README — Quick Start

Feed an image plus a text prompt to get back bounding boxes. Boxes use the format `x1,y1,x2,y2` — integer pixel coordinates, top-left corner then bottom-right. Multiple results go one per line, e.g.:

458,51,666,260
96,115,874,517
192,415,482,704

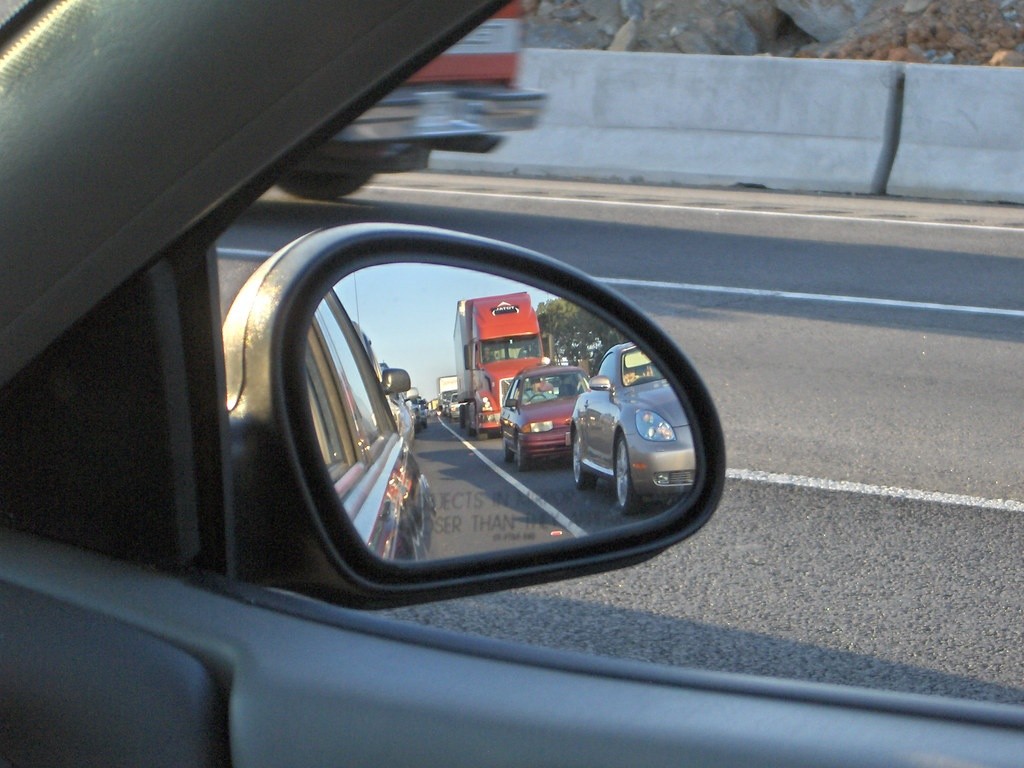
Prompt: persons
624,365,653,384
482,348,495,363
523,377,558,405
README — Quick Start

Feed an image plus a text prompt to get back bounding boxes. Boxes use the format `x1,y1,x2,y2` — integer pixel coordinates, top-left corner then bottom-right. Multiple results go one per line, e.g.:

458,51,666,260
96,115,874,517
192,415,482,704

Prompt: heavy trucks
453,290,546,439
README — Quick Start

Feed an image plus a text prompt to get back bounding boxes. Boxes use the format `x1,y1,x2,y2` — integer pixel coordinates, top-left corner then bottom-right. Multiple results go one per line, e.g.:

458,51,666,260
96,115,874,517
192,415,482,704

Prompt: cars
352,321,429,451
568,343,696,517
304,283,439,564
443,394,467,423
498,367,594,471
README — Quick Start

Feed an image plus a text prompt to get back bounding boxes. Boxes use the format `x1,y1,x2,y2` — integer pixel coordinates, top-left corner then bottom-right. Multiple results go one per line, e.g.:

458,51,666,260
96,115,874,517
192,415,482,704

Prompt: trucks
436,375,457,412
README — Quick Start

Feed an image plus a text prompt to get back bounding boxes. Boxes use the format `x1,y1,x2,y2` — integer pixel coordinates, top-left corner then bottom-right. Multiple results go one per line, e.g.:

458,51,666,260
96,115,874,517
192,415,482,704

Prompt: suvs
278,1,552,199
